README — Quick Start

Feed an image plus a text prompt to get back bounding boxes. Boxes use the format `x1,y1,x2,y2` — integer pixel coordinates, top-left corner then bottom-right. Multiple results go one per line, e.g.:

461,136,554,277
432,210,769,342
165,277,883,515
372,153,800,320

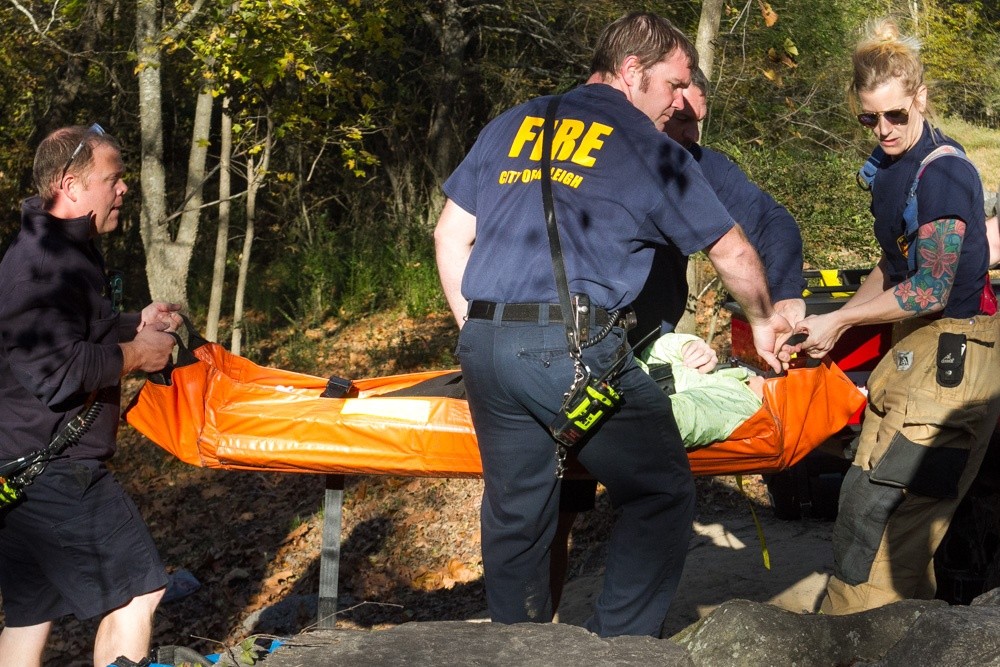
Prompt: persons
432,10,805,639
792,19,1000,616
1,127,184,667
365,332,766,448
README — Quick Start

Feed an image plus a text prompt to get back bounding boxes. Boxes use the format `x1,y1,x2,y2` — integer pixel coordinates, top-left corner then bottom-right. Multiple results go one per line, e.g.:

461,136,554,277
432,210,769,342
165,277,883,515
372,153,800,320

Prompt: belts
467,300,611,326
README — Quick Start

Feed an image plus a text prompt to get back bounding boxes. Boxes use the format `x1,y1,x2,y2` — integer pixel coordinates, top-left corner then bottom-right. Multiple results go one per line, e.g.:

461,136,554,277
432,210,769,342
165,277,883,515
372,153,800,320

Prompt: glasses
856,91,918,129
59,123,105,189
